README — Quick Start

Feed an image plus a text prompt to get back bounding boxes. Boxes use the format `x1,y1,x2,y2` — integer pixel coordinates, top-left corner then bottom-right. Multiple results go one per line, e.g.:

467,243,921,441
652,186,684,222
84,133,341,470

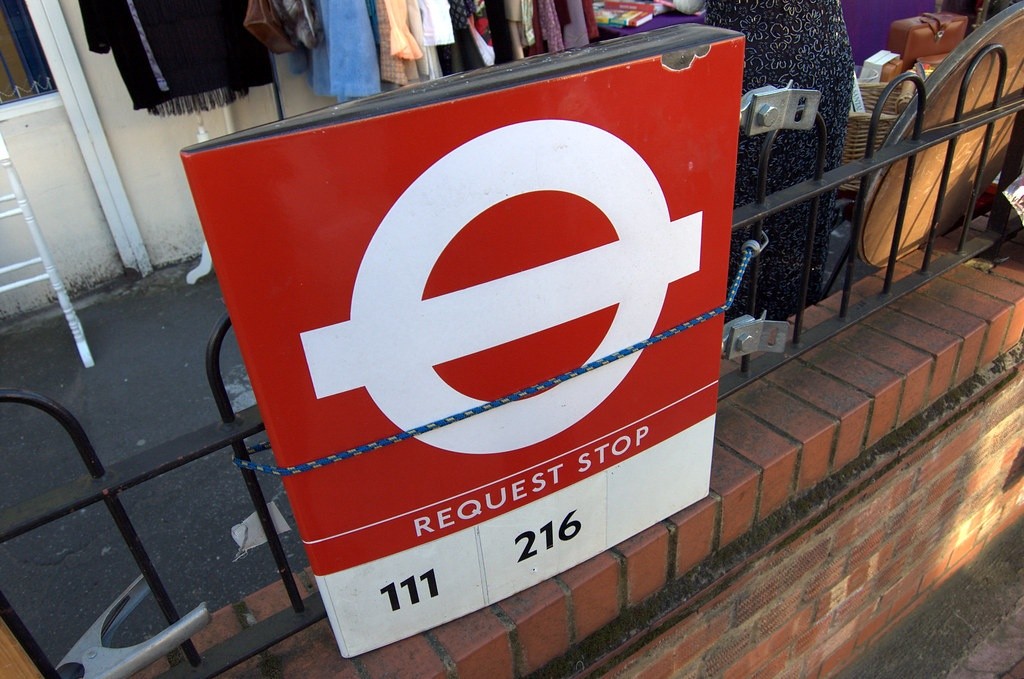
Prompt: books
592,0,706,28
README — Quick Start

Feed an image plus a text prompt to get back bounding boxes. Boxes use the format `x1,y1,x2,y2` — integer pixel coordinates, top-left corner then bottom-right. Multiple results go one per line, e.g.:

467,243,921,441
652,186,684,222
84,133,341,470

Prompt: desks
598,10,705,38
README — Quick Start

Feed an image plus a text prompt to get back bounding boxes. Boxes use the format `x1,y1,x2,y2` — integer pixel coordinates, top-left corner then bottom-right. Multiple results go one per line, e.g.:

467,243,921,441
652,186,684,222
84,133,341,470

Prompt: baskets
838,79,909,195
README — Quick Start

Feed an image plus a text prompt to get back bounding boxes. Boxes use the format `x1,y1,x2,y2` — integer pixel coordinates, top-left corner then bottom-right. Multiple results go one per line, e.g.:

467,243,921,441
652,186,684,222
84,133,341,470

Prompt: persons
672,0,855,321
